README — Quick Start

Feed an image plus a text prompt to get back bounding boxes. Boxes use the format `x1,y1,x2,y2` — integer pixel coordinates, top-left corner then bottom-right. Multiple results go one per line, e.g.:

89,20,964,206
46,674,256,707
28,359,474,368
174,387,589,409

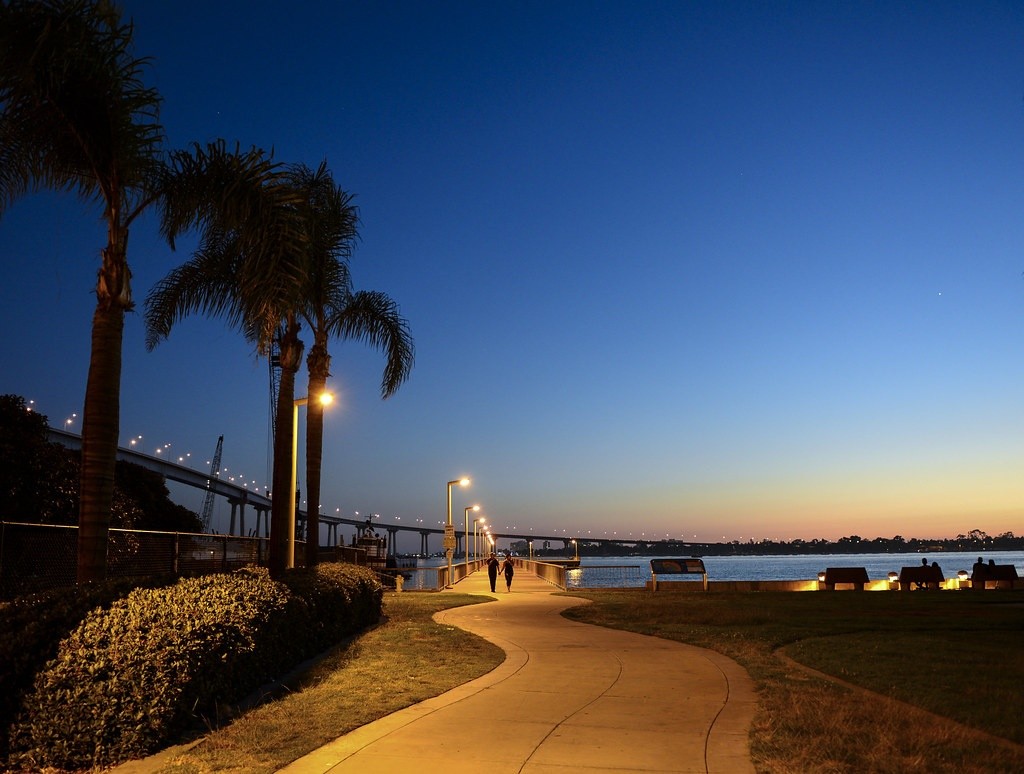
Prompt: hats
488,552,495,555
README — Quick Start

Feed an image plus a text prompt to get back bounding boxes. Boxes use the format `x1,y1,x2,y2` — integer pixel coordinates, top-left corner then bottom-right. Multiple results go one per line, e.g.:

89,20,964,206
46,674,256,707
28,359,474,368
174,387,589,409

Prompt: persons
498,553,514,592
486,552,501,593
971,557,999,589
914,558,939,591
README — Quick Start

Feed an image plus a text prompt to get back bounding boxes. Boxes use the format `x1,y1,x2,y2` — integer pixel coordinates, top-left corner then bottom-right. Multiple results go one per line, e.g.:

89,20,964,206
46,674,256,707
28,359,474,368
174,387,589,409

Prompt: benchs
967,564,1019,589
893,566,945,590
824,567,870,590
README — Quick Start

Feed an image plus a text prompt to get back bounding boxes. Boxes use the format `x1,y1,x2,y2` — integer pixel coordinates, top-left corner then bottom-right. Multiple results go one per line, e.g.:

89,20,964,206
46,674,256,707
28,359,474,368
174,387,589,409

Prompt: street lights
529,541,532,560
572,540,577,560
464,506,480,577
473,517,486,572
478,526,494,572
442,476,469,590
284,393,334,569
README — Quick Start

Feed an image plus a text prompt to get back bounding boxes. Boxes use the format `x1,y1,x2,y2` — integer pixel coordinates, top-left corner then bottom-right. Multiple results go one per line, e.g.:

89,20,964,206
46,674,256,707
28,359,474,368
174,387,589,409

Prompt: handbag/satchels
512,566,515,574
